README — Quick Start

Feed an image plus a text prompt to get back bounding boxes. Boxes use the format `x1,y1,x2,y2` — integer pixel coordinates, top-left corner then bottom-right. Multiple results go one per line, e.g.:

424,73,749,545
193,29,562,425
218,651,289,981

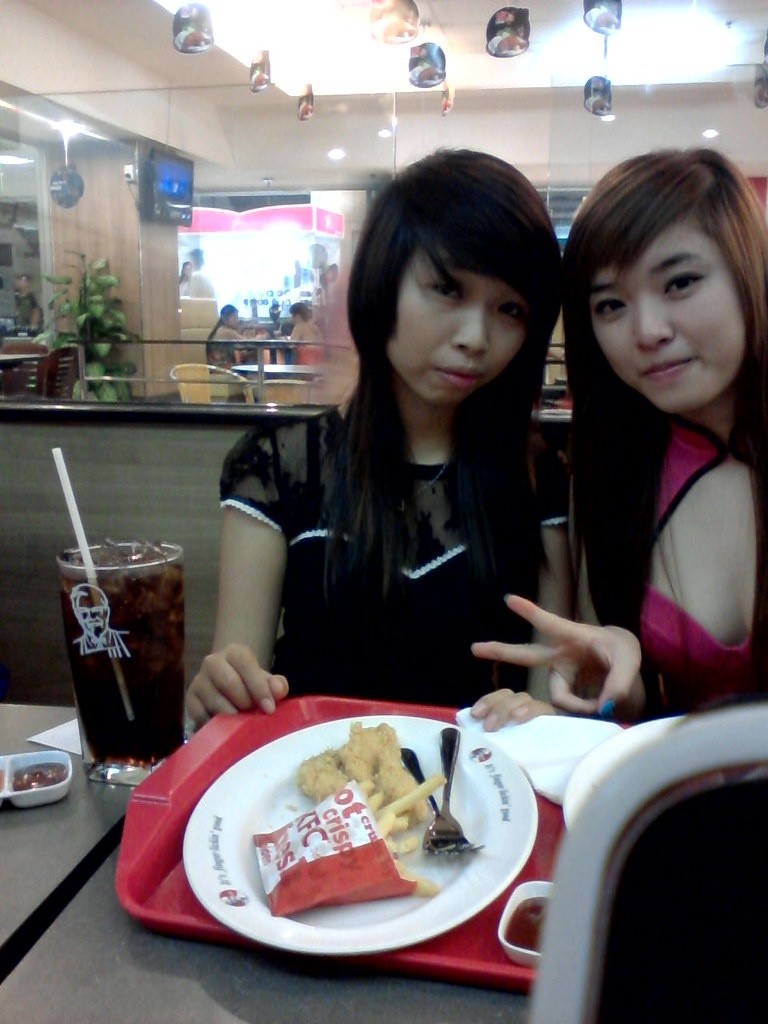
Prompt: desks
0,701,534,1024
232,363,320,379
0,353,47,365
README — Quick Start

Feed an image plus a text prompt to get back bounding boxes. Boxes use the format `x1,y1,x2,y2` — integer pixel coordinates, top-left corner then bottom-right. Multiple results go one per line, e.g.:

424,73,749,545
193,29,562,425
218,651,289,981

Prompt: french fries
361,771,447,897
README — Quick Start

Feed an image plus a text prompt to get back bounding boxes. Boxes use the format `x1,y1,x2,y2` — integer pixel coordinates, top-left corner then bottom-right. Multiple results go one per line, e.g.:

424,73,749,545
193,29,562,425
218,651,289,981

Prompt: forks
401,748,485,852
422,728,465,858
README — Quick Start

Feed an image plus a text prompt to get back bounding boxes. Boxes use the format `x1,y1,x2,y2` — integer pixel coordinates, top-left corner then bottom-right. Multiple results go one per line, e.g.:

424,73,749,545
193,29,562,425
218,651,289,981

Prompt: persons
180,149,572,733
15,275,38,324
470,141,768,717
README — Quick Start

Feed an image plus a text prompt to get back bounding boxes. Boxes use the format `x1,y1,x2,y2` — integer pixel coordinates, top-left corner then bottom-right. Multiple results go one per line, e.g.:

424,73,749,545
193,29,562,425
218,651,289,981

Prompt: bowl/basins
0,750,72,808
498,882,552,966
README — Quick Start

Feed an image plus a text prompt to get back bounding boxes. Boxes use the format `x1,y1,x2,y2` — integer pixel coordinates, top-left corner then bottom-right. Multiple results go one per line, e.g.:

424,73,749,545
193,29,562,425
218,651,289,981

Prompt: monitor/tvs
140,146,195,227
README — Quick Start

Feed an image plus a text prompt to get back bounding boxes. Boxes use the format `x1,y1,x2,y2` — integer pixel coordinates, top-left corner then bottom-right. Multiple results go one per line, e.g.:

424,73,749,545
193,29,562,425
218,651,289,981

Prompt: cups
56,542,186,785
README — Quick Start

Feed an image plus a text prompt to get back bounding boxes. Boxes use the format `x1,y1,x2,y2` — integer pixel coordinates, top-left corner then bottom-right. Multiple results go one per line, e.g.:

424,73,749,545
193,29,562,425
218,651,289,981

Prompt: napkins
454,703,626,807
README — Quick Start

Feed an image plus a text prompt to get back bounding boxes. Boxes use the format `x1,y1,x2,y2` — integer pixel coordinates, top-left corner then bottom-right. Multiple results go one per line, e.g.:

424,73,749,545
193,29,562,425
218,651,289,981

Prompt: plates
182,716,538,955
563,716,682,826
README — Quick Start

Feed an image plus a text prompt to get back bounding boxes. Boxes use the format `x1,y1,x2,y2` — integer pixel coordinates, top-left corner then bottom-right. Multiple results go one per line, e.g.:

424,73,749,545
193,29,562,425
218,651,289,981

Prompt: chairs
170,364,311,405
0,343,80,399
